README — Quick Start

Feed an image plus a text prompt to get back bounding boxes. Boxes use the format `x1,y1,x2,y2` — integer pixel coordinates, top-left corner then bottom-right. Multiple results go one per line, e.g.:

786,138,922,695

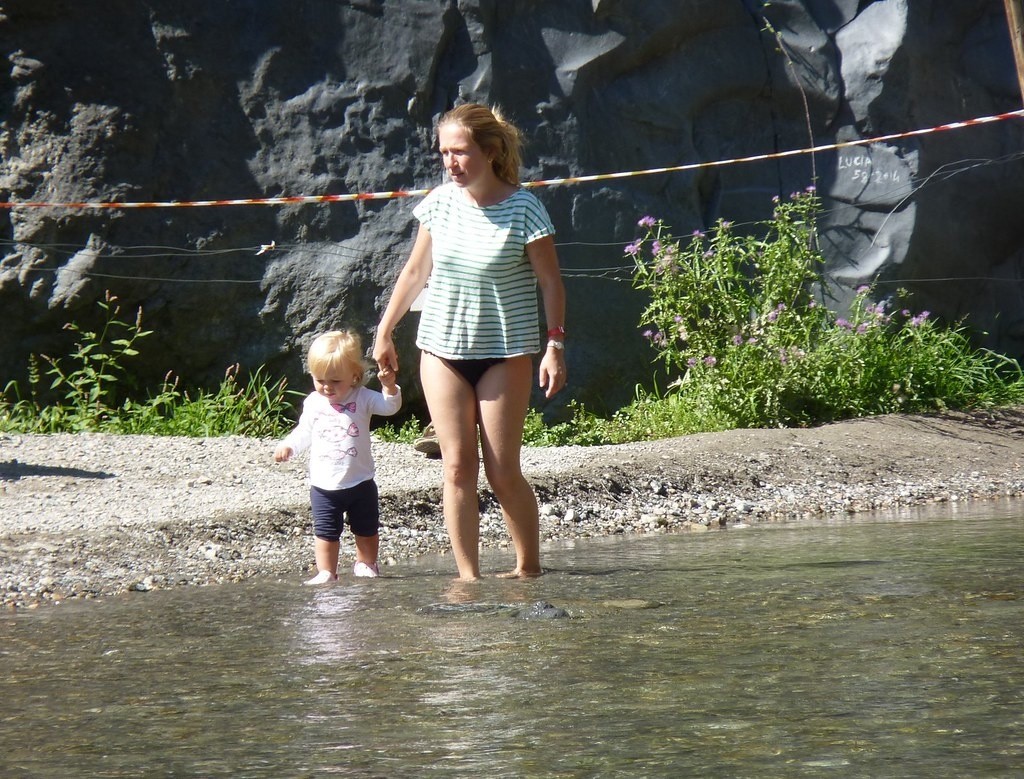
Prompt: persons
274,328,403,586
372,103,567,582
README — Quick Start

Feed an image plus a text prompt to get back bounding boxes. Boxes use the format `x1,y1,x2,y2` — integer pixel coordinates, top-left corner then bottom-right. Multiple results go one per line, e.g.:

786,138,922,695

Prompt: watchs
547,341,565,352
548,325,565,336
